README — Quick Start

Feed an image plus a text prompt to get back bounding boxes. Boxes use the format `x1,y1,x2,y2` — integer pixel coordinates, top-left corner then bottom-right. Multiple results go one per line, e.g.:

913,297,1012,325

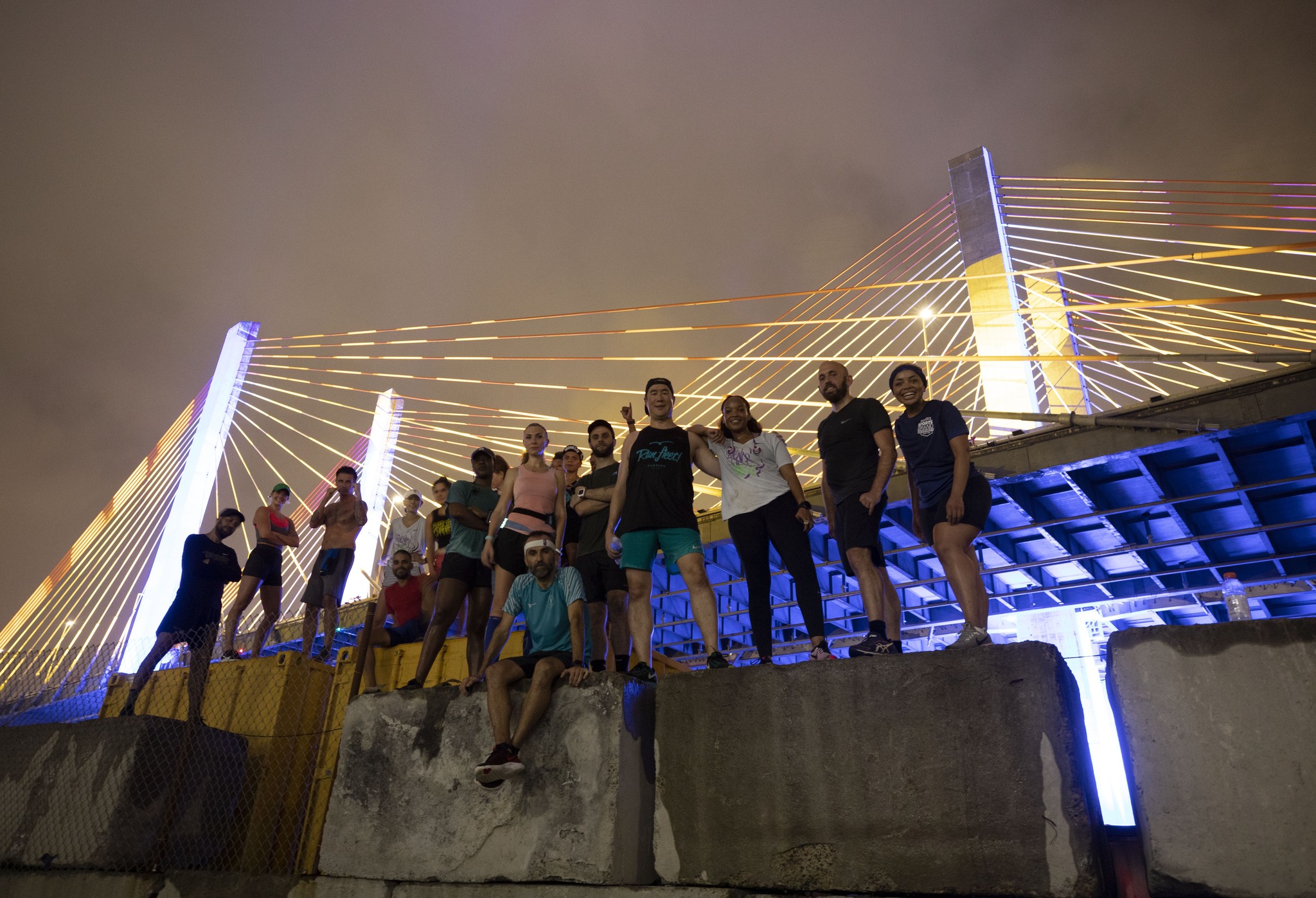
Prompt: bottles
610,537,623,565
1221,572,1252,622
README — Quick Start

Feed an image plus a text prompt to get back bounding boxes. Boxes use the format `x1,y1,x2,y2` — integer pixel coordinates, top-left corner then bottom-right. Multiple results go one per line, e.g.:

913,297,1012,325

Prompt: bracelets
424,557,428,563
555,549,563,556
485,535,494,541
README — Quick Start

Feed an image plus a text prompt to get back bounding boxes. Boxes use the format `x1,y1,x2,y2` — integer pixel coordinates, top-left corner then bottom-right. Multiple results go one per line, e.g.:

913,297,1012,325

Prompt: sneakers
809,635,837,661
310,645,333,664
619,660,658,685
751,656,773,665
392,678,423,692
945,621,994,651
219,649,246,662
848,632,900,658
705,644,736,669
473,741,526,791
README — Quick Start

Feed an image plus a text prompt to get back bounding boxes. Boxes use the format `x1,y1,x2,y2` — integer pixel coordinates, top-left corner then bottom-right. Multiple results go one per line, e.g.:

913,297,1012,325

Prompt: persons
568,419,631,675
380,489,427,590
397,447,500,691
459,530,593,791
589,453,597,473
480,422,567,681
299,465,368,663
423,476,467,638
551,451,567,475
219,483,300,662
604,378,787,678
888,362,992,650
361,548,438,693
557,445,584,567
119,507,245,725
817,362,903,657
686,395,838,663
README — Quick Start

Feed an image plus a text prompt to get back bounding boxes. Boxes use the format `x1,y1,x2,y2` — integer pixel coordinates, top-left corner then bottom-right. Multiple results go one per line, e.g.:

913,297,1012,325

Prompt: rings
804,520,809,525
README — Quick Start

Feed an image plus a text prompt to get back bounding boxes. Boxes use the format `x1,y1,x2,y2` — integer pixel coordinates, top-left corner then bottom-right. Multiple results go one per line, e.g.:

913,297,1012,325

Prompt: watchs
798,501,811,510
578,488,588,500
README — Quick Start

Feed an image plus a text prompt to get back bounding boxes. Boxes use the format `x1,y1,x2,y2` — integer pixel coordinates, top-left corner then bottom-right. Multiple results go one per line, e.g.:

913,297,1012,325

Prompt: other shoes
119,704,135,717
347,687,381,705
186,715,209,728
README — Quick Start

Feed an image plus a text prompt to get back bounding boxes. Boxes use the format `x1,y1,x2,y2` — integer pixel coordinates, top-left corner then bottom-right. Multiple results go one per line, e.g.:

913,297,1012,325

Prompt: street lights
920,309,934,401
373,495,400,595
45,620,74,683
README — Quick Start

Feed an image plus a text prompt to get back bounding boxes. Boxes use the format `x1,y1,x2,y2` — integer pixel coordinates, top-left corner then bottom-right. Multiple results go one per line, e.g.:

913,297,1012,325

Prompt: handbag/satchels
431,678,463,689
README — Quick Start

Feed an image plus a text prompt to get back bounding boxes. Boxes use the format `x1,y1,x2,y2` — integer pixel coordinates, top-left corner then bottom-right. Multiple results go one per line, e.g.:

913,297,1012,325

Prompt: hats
564,445,585,463
587,419,613,435
644,378,673,417
471,447,495,459
405,489,422,501
271,483,291,497
219,508,245,522
